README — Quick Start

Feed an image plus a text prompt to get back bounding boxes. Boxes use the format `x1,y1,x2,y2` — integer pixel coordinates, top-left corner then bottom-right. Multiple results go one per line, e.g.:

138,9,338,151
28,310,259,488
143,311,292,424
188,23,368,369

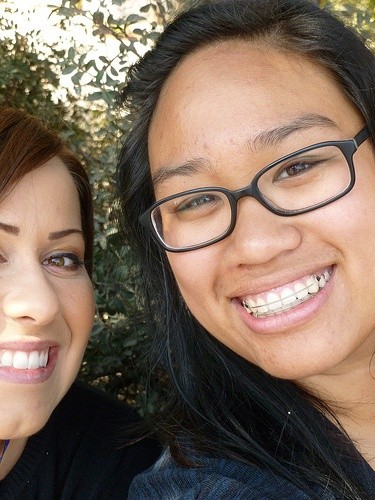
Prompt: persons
0,105,166,500
114,0,375,500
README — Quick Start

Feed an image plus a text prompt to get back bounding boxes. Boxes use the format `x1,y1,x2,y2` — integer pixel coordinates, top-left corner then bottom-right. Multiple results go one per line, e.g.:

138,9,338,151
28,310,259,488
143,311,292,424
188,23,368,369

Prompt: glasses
137,124,375,253
